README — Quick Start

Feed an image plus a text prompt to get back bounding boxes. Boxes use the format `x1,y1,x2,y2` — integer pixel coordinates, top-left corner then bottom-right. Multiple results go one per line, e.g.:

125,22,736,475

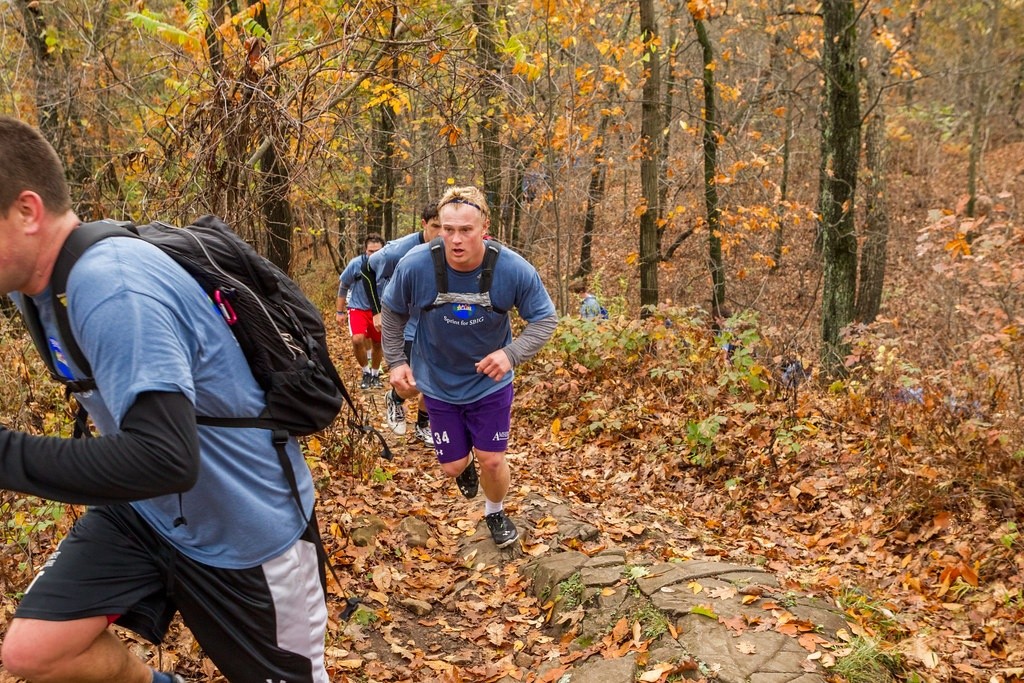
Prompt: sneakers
383,389,407,436
413,421,435,447
483,510,519,549
371,374,383,389
361,371,372,388
456,448,480,500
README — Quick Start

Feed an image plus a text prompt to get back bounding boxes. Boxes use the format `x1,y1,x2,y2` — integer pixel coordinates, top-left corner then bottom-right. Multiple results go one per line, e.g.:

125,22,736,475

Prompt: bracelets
337,311,344,315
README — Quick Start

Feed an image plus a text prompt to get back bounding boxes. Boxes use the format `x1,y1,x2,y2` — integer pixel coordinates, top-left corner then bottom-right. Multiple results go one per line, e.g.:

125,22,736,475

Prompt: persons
335,232,386,388
575,287,602,320
380,186,558,549
367,199,446,447
712,305,739,352
0,118,331,683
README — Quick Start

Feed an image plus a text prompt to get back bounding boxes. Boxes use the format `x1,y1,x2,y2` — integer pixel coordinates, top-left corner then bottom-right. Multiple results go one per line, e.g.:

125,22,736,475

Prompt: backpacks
20,214,393,623
